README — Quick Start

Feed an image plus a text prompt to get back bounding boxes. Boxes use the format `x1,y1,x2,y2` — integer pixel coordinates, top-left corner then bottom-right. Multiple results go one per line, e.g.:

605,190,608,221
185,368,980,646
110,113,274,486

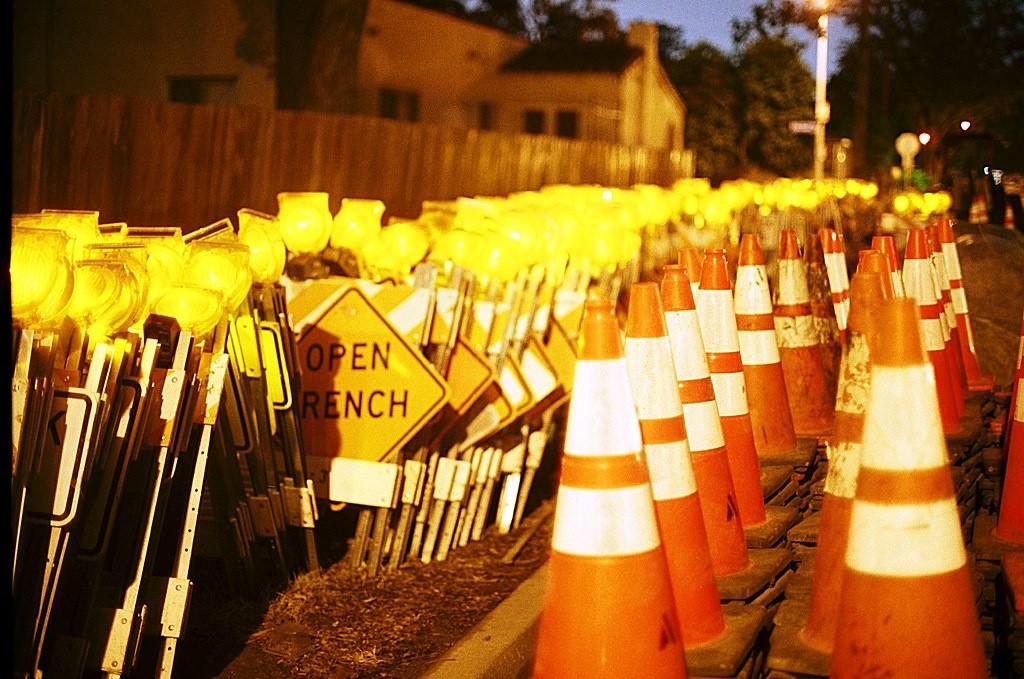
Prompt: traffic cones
817,298,988,678
978,193,991,225
621,279,769,678
660,266,796,604
991,344,1024,547
970,197,982,226
532,300,693,679
1003,204,1017,231
680,212,993,438
778,271,997,676
